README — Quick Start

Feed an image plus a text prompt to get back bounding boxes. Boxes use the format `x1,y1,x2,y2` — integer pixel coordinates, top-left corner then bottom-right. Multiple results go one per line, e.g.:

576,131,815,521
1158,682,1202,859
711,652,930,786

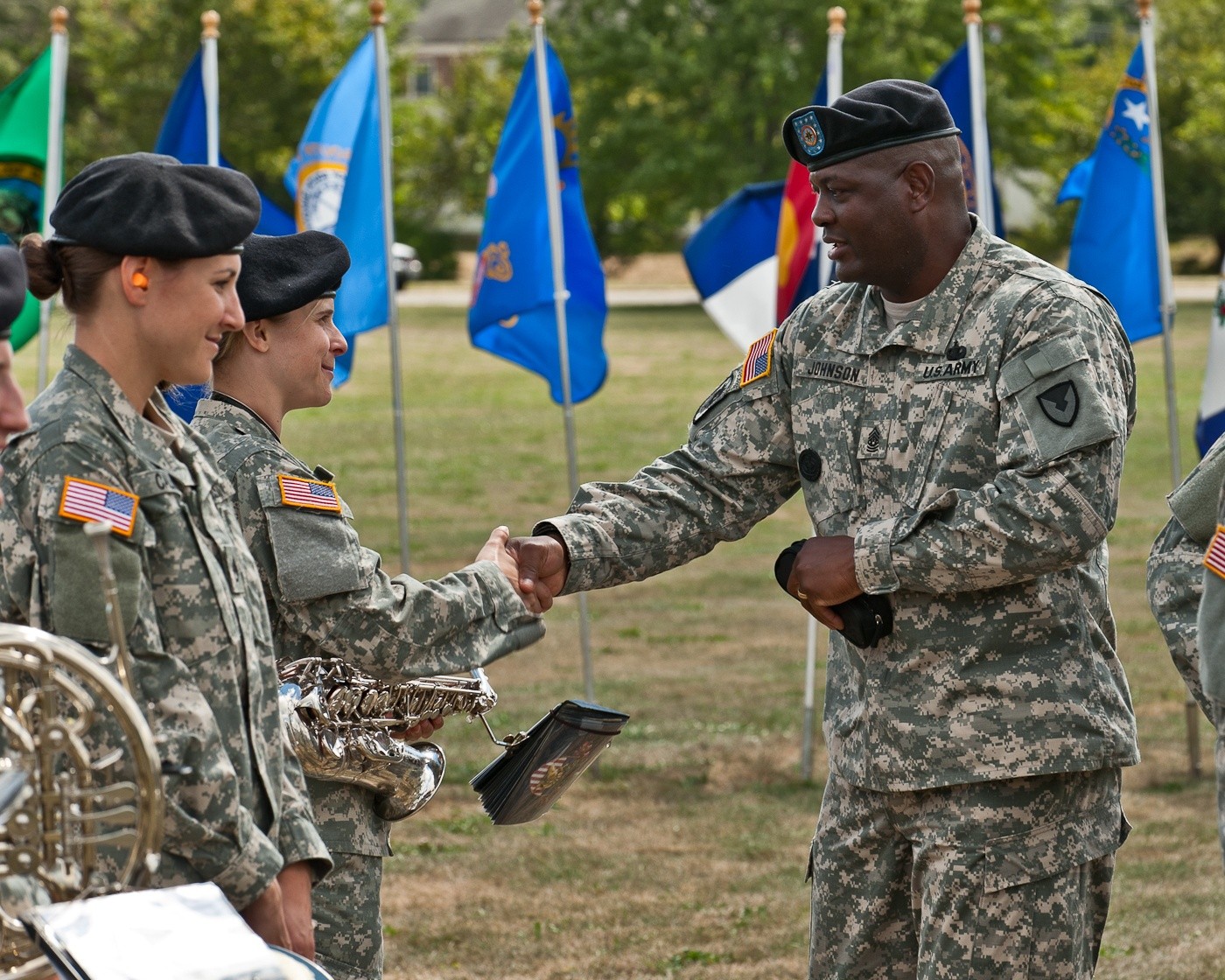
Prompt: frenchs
1,520,163,980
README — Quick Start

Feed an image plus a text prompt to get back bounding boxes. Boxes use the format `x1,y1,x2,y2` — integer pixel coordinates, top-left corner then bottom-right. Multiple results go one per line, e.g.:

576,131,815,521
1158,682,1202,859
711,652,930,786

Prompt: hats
0,245,26,338
782,78,962,171
46,151,258,259
237,232,351,322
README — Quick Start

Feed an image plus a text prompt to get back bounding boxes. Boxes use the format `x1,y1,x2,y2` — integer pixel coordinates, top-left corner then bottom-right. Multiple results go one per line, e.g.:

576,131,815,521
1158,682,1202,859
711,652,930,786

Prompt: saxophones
282,659,501,823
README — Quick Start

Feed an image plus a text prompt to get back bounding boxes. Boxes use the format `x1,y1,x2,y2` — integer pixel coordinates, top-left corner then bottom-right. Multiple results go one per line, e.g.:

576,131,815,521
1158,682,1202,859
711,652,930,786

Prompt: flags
1057,44,1178,342
930,43,1006,239
284,30,392,390
776,72,841,327
155,44,297,425
466,39,610,405
0,33,68,353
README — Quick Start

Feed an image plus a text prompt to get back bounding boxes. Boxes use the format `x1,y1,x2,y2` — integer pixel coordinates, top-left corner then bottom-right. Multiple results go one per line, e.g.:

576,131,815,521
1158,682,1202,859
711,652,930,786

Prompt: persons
0,151,334,980
188,231,551,980
1148,431,1225,875
504,75,1141,980
